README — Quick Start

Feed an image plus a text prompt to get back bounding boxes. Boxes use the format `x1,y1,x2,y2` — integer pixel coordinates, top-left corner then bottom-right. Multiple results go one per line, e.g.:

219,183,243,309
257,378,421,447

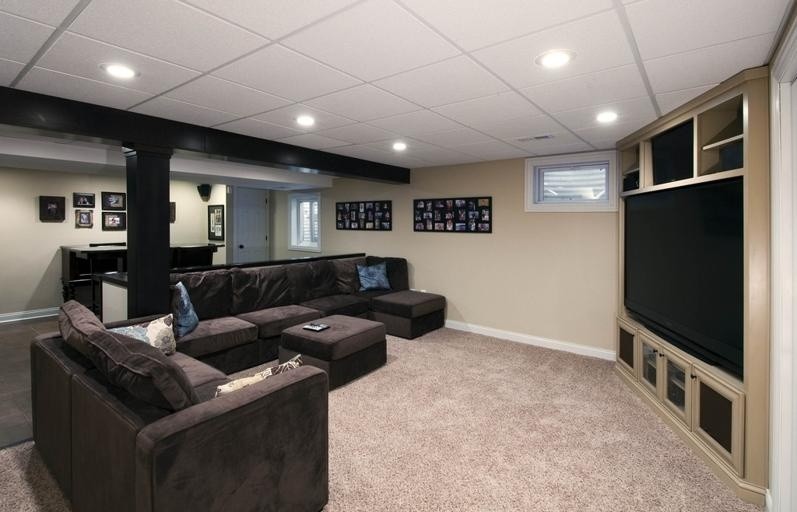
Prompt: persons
84,196,91,205
336,202,392,229
47,200,58,215
416,198,490,232
78,195,84,205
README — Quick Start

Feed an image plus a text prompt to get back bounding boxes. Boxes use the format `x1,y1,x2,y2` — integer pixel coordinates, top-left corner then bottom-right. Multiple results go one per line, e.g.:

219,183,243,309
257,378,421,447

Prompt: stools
371,289,448,340
277,313,392,392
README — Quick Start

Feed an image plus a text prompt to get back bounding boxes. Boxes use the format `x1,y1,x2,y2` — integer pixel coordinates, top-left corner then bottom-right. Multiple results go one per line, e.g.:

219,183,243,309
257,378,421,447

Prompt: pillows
169,281,199,339
53,296,104,350
213,353,305,399
353,261,391,292
97,314,177,359
84,327,205,409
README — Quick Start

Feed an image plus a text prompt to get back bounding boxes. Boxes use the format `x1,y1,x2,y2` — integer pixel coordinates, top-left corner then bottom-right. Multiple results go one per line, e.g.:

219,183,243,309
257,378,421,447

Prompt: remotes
302,324,322,332
310,322,329,329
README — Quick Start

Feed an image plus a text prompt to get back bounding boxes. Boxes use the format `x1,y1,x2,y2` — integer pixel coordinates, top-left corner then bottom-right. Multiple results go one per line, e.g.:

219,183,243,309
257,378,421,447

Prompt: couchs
26,314,331,510
168,253,413,384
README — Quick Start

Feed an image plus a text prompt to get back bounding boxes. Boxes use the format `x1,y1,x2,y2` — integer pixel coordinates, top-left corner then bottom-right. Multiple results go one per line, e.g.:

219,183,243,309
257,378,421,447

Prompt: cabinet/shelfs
59,242,219,318
613,62,769,510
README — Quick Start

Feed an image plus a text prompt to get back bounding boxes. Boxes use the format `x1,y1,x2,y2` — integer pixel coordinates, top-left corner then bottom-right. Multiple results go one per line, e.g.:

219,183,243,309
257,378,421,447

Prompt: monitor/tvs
623,176,744,383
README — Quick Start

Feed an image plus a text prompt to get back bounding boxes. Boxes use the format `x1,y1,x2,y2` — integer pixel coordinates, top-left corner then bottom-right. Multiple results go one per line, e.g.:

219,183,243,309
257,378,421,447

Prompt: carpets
0,328,767,510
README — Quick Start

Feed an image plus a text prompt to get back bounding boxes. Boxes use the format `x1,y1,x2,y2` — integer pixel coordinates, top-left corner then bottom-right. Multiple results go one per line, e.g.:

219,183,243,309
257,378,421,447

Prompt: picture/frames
206,204,224,241
39,194,65,222
74,209,92,227
73,191,95,208
411,197,493,234
101,212,126,231
101,191,126,211
334,200,391,231
